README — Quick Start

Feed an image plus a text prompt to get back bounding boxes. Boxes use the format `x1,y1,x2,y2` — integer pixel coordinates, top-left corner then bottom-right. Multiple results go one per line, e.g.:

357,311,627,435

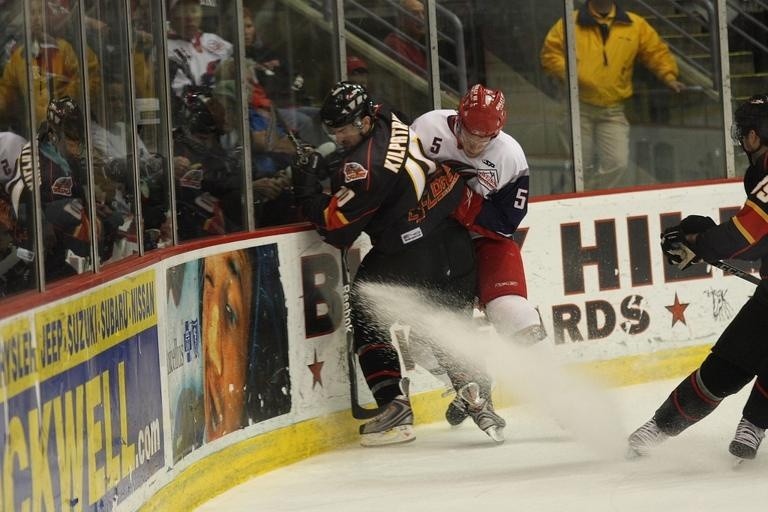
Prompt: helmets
47,95,84,141
458,84,507,138
179,84,230,138
731,94,768,142
320,80,376,128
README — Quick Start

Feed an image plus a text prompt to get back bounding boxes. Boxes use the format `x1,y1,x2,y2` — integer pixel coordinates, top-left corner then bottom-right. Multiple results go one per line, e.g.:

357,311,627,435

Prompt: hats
348,56,366,76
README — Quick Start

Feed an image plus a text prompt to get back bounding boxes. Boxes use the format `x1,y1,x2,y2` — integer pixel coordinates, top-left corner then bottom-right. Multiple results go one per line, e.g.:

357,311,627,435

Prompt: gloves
291,144,330,198
660,214,718,271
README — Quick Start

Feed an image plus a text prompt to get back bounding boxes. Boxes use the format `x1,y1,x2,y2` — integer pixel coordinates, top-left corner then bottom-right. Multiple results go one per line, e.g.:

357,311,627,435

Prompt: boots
359,376,413,434
445,373,505,431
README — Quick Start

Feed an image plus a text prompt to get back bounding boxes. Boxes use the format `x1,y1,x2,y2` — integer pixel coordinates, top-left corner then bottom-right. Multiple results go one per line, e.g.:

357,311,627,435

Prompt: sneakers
729,416,765,459
628,418,667,456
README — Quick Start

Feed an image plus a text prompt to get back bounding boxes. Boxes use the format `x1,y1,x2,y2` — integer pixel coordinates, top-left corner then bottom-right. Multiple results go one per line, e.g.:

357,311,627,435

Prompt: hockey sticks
341,248,391,420
254,67,303,158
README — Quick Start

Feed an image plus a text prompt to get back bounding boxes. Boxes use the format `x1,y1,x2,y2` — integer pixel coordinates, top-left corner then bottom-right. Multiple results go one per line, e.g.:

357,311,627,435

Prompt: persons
195,243,298,447
624,92,767,462
407,82,585,442
537,0,690,196
292,79,511,433
0,2,430,299
